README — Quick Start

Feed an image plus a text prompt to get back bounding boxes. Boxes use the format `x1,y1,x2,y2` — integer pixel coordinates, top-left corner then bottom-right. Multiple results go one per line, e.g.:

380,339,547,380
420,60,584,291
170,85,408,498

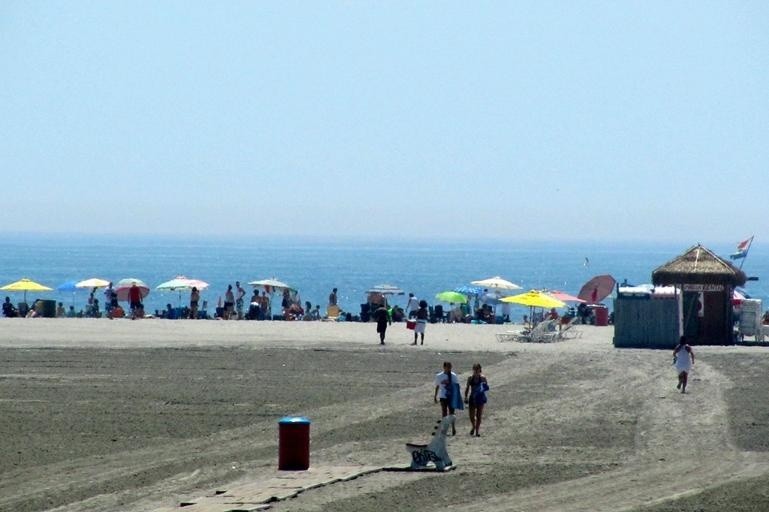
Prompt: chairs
406,414,457,473
497,314,587,342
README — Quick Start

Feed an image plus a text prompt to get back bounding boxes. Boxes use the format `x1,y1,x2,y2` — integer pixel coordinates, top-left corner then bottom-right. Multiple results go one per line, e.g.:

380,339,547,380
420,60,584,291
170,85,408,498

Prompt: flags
728,233,755,261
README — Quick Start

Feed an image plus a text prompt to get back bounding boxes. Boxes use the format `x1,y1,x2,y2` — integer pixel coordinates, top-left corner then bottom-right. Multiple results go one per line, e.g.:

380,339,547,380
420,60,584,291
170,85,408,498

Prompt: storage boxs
406,317,417,329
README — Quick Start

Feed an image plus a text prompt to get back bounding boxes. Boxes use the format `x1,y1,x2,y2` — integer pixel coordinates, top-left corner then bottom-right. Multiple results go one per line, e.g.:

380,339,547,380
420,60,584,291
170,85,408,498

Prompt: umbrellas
546,290,587,304
497,289,571,334
577,273,617,305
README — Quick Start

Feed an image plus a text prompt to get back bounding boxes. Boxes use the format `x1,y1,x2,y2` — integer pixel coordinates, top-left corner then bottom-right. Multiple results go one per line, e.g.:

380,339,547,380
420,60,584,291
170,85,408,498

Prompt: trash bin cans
595,308,608,326
279,418,311,470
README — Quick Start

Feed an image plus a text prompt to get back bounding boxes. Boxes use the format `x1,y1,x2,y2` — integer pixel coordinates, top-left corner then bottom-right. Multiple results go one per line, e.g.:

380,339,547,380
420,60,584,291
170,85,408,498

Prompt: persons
464,362,490,437
673,333,696,393
432,359,464,438
408,299,428,346
522,307,576,324
373,303,391,345
